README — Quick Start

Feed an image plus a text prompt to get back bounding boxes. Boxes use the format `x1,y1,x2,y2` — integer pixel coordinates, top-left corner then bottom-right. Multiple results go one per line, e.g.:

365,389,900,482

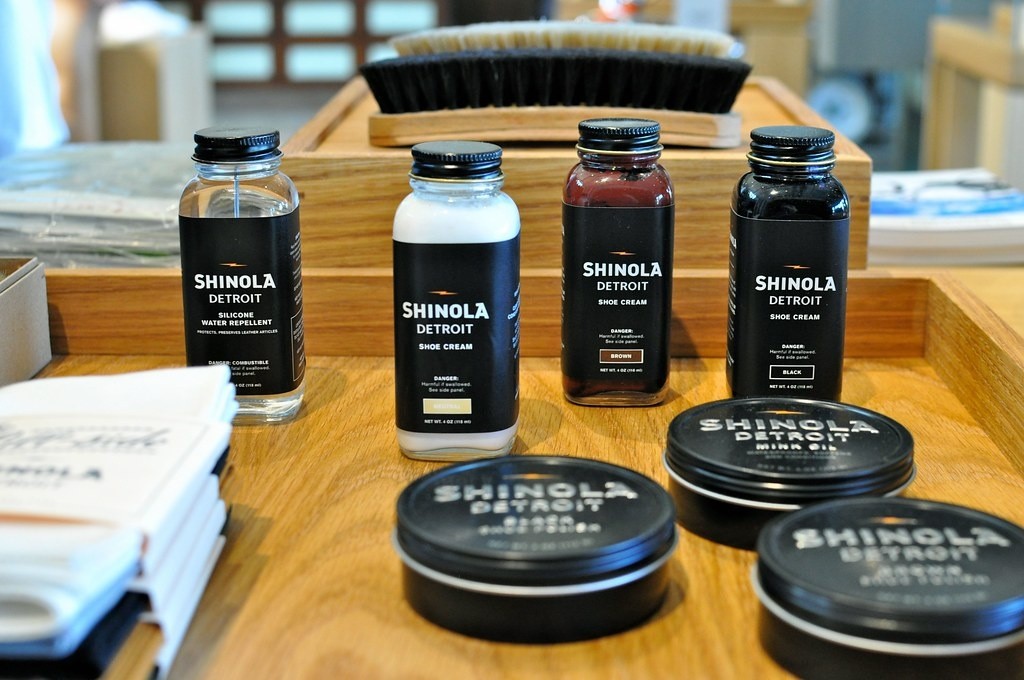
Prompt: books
866,168,1024,267
0,366,239,680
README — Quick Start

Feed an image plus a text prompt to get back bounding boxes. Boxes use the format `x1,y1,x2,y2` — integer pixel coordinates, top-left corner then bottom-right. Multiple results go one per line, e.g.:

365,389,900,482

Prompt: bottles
178,126,306,424
726,125,850,404
392,139,521,461
560,117,675,406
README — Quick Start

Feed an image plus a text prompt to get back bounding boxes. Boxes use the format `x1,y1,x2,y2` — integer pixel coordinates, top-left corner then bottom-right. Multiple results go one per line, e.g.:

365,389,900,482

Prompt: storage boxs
0,256,52,389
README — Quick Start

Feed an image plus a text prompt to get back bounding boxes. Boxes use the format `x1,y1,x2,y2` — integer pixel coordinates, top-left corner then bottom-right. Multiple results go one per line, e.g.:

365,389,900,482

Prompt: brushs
357,19,754,151
